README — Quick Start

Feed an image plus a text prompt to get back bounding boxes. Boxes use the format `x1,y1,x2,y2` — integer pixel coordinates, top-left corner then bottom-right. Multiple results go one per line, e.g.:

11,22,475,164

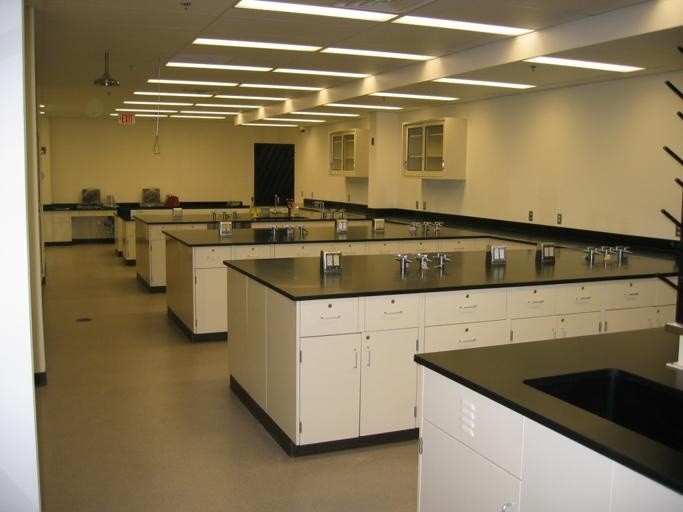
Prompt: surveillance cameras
299,127,306,134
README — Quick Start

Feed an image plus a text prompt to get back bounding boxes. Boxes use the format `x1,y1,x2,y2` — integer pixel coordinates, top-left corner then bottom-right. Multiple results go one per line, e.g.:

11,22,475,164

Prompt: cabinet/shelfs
400,117,466,180
327,128,367,178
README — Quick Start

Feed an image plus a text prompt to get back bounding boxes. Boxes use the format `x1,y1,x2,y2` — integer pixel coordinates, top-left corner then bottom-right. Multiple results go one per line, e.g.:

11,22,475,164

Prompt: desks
42,204,116,246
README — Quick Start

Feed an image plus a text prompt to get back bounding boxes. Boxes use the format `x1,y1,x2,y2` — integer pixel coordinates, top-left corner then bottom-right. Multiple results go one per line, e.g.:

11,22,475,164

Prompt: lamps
93,49,120,88
